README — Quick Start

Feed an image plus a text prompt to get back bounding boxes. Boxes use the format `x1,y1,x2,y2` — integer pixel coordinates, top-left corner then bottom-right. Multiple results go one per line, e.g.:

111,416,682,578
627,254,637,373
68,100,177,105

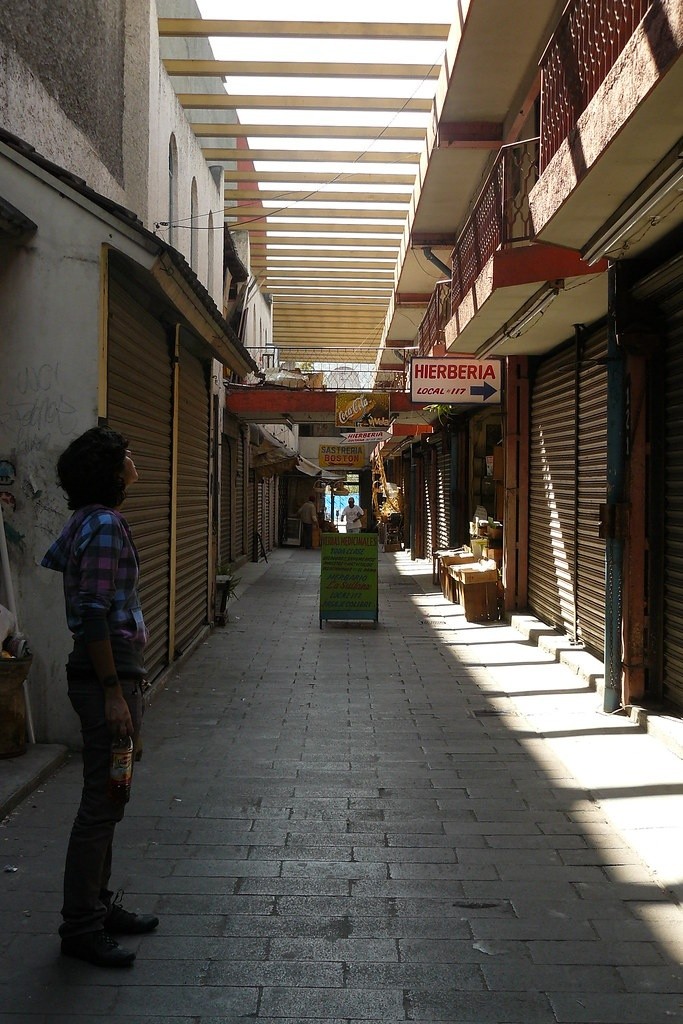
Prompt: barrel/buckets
0,654,34,758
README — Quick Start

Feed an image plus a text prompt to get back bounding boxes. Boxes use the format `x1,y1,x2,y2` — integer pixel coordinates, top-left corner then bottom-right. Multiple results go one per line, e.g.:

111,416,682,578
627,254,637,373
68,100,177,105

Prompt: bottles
109,736,133,806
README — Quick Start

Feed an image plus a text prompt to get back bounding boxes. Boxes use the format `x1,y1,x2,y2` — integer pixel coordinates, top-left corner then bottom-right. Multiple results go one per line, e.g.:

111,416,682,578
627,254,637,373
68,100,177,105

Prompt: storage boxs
438,519,503,624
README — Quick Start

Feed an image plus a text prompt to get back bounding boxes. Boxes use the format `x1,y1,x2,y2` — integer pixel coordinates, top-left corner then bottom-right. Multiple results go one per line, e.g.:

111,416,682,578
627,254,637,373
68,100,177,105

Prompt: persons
341,497,364,533
41,427,158,969
297,495,319,549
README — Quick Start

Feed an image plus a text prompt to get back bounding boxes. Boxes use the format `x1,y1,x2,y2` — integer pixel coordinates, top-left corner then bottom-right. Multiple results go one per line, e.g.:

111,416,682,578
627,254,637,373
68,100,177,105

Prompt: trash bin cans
0,654,33,759
215,574,232,617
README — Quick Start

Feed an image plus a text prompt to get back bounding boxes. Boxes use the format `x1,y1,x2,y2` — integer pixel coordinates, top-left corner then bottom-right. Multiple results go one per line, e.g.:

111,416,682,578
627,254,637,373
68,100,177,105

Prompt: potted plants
215,563,242,616
303,356,324,389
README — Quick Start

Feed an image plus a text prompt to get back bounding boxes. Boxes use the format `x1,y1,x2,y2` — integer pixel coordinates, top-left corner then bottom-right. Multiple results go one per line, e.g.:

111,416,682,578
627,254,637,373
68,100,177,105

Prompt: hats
349,498,354,503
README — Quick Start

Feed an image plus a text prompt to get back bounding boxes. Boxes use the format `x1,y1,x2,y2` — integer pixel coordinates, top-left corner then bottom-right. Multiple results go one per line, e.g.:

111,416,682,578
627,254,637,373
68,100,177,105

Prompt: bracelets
99,672,118,688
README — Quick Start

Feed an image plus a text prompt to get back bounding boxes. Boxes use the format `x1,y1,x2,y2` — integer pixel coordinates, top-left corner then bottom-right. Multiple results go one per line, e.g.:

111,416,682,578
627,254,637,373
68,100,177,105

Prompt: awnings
255,440,301,482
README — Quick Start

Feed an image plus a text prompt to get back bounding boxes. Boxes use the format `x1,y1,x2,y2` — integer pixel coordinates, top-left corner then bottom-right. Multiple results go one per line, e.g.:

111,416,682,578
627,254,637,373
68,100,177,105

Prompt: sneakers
105,889,159,934
60,931,136,967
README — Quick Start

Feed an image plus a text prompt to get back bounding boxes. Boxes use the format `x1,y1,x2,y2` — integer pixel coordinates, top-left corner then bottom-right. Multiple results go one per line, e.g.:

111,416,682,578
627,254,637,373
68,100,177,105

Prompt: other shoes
305,546,313,549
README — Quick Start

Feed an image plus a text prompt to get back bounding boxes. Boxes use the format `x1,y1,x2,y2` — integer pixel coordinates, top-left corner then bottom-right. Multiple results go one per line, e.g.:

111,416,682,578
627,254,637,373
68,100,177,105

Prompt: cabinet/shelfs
281,516,304,548
